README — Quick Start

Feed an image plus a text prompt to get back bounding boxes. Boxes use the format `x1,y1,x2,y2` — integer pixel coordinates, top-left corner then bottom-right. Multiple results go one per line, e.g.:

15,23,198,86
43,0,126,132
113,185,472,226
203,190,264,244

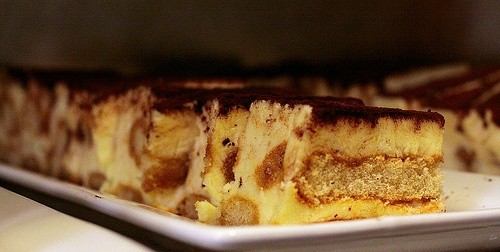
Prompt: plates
0,161,496,252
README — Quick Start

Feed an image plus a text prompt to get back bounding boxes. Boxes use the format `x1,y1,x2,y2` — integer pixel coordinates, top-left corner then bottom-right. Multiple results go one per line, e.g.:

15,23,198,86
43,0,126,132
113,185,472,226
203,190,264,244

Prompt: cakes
0,68,500,228
